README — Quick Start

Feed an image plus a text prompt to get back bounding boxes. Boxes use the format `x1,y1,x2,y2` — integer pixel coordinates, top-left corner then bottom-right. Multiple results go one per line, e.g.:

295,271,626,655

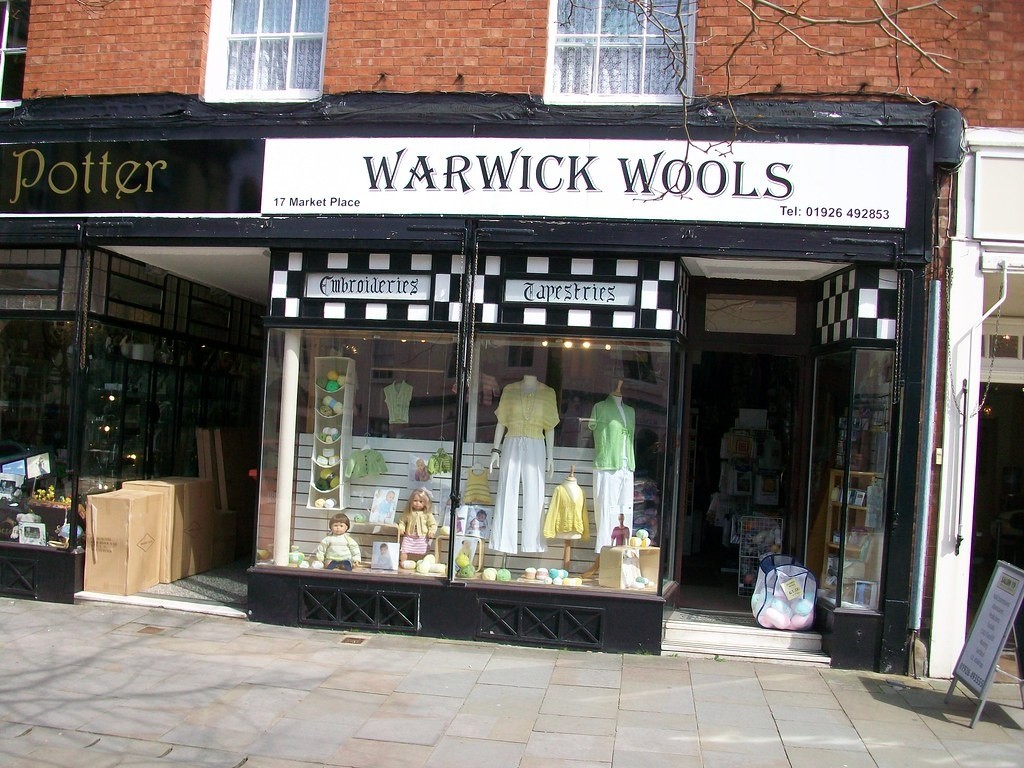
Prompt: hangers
359,432,372,452
460,455,490,476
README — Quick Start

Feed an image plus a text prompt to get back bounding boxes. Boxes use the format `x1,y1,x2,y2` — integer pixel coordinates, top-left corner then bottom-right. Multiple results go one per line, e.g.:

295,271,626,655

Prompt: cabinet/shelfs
305,357,357,511
817,390,891,615
599,545,660,593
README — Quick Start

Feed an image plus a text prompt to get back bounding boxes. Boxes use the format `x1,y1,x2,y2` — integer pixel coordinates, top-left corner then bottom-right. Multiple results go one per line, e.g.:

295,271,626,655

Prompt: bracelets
490,448,501,454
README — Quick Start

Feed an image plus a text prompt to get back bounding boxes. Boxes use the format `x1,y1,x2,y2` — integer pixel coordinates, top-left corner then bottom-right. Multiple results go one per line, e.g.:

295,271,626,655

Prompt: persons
489,375,561,554
465,510,488,534
610,513,630,546
398,487,437,567
375,491,396,524
378,543,393,569
315,513,361,571
543,476,590,541
415,459,429,481
762,476,778,492
589,392,637,555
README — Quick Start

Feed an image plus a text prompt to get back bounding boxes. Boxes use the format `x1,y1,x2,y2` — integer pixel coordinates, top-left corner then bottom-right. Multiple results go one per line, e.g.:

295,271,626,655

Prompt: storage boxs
84,490,160,595
122,476,220,584
737,515,784,594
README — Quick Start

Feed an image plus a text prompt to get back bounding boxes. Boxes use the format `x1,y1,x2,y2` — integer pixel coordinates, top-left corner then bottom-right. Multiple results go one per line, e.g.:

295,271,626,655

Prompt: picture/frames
18,523,45,546
